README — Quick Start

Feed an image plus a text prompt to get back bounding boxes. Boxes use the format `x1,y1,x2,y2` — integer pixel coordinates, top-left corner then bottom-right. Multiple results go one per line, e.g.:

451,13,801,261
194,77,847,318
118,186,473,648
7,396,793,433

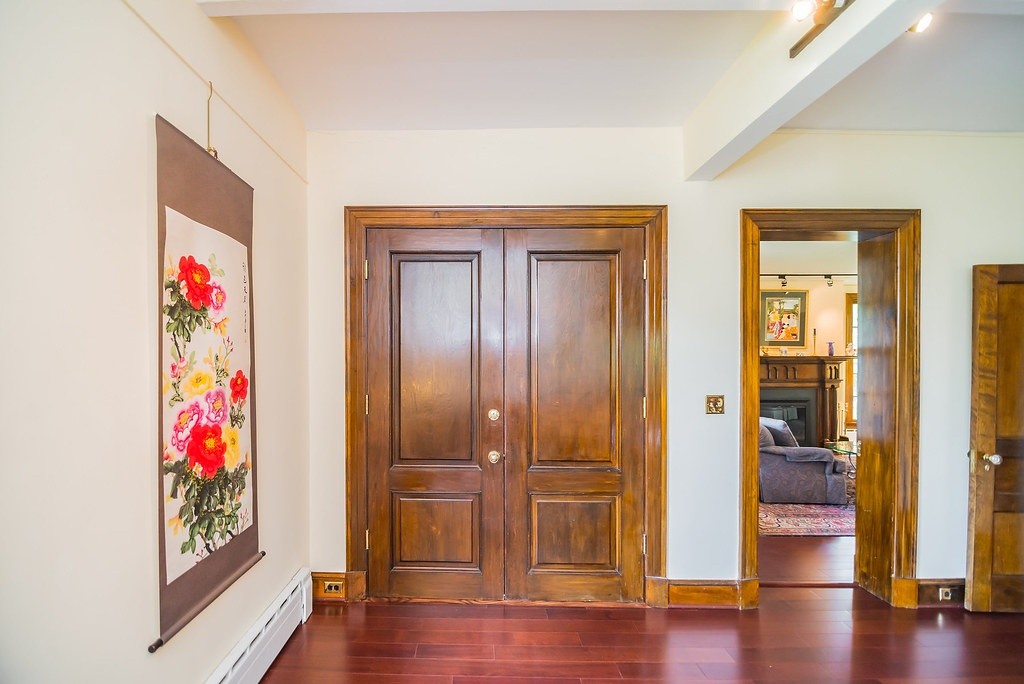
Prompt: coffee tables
828,440,857,479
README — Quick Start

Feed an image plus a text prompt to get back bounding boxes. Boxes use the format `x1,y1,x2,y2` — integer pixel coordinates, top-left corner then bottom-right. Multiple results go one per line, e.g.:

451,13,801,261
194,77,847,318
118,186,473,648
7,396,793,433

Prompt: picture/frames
760,289,809,350
148,113,265,654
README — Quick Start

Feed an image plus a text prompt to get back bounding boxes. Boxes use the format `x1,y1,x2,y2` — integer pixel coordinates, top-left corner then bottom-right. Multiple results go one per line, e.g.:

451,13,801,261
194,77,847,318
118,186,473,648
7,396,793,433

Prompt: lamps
778,276,788,286
908,12,934,33
825,275,834,287
790,0,849,25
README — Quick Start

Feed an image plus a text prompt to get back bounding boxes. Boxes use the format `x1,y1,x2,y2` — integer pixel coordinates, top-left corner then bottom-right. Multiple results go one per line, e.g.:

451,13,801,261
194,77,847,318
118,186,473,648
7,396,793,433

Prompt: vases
828,342,834,356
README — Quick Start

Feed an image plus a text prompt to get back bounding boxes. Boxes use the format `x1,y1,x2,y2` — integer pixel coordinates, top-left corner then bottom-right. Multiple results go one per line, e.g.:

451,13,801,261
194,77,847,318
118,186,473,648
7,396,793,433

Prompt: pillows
759,416,800,447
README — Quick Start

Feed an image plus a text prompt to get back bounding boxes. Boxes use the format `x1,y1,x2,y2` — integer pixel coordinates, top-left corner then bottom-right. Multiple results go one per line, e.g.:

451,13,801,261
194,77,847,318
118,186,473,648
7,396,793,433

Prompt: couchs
758,424,848,505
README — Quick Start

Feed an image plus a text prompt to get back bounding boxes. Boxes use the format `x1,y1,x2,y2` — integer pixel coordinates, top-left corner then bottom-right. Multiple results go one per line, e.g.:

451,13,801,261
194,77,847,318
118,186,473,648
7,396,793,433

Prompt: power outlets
324,582,343,593
939,588,951,601
